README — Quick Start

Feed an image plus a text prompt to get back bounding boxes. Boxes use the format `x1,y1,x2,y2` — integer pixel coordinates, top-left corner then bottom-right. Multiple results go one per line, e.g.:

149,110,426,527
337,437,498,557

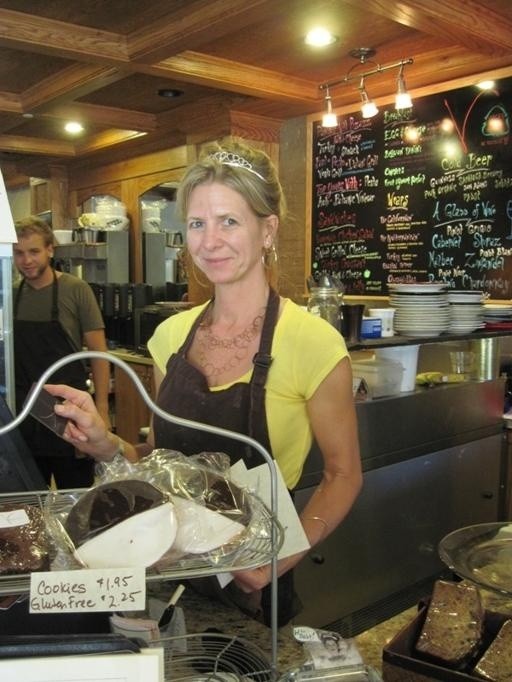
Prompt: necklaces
197,315,265,378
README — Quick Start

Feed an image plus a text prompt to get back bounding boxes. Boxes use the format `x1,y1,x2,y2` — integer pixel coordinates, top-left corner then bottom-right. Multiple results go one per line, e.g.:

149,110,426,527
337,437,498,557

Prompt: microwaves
132,306,184,351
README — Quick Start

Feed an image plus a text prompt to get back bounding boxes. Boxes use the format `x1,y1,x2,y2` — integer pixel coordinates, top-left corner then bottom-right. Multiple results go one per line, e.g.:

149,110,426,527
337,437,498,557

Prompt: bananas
416,371,442,386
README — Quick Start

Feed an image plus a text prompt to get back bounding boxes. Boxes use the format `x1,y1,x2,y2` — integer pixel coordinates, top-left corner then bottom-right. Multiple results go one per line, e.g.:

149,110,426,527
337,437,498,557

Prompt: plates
384,281,511,338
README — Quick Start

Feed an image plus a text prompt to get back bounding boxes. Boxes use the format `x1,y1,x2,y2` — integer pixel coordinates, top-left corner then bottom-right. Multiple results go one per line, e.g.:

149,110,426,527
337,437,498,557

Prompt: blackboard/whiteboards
301,66,512,305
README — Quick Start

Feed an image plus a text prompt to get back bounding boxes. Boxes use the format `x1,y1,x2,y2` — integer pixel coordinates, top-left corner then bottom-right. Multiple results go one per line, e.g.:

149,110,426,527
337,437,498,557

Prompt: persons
41,140,364,638
12,215,113,489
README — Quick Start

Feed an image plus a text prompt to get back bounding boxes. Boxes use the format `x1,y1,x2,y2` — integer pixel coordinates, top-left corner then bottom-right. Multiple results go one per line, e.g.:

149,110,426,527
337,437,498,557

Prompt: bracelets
300,517,329,542
111,438,125,464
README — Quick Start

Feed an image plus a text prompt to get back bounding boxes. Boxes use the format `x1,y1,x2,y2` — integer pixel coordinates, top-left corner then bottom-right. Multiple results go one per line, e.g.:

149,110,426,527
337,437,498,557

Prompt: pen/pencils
158,584,186,633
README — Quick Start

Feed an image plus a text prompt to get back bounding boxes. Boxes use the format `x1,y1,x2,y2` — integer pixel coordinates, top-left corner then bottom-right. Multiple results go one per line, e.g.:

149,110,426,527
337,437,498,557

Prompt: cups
360,314,382,338
447,352,477,382
468,337,501,383
369,306,398,338
338,303,365,343
107,600,177,642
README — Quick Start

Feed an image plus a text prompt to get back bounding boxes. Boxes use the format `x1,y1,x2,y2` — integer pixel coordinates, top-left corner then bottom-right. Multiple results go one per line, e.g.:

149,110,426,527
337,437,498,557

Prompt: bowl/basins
436,521,511,597
52,230,73,244
72,228,104,244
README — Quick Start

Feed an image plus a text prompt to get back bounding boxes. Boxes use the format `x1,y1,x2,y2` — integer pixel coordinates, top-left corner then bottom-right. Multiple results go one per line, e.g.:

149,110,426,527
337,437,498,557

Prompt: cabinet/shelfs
0,488,278,682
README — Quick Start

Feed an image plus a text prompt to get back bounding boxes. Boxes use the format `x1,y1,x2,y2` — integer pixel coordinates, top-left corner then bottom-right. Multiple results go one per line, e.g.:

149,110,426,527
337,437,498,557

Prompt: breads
0,503,51,571
415,578,511,680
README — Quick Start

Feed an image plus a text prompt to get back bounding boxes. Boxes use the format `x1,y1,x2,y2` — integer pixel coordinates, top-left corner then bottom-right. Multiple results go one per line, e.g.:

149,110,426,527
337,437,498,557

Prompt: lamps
317,46,413,128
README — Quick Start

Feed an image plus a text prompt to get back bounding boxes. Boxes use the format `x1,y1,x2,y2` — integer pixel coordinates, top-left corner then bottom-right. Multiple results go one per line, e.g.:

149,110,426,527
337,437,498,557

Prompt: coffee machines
86,282,156,350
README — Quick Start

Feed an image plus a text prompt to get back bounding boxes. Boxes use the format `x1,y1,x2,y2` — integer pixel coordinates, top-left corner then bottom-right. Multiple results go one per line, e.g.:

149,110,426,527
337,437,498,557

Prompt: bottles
307,285,341,332
